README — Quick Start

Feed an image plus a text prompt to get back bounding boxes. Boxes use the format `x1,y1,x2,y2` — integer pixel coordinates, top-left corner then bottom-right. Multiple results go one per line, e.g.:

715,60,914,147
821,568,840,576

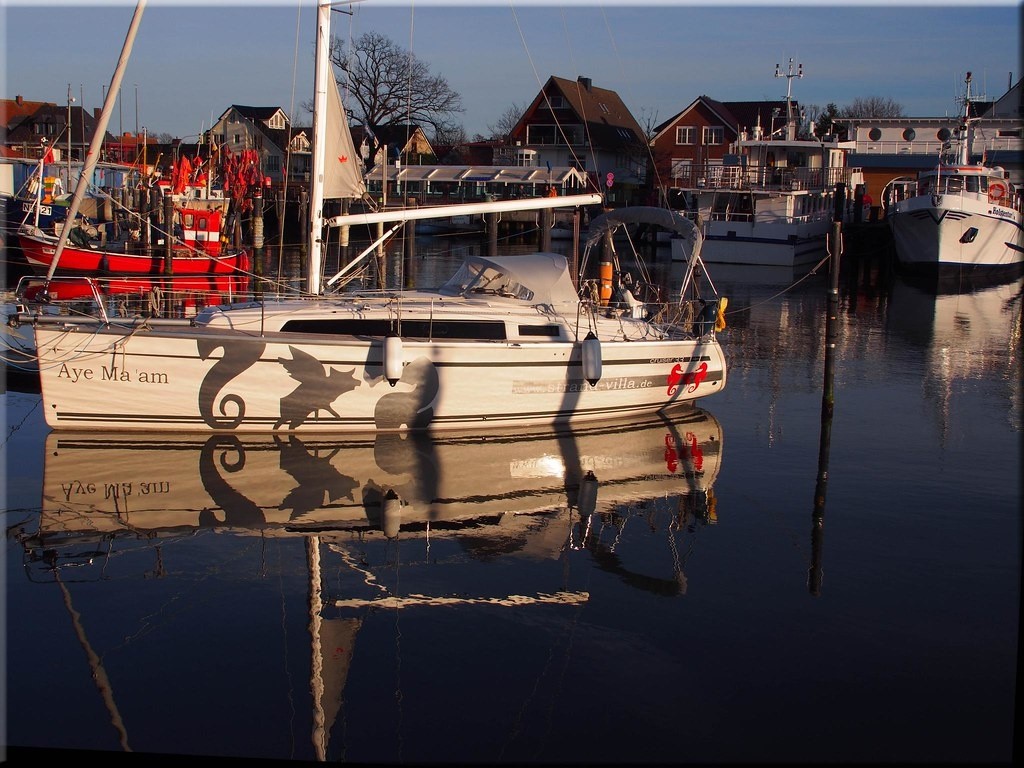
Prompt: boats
670,58,872,268
18,82,250,279
884,71,1024,280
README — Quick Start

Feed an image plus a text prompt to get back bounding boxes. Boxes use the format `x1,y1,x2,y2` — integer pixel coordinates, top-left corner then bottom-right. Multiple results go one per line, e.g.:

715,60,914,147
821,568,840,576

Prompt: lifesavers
989,183,1006,200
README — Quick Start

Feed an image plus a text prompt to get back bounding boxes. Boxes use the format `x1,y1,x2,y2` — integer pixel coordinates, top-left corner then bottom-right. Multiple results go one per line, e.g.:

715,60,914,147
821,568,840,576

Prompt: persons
516,185,527,199
541,186,557,197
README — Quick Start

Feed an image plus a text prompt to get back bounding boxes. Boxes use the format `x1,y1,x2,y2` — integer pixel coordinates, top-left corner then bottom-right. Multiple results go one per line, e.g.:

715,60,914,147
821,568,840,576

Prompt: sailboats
7,404,724,762
1,3,729,432
